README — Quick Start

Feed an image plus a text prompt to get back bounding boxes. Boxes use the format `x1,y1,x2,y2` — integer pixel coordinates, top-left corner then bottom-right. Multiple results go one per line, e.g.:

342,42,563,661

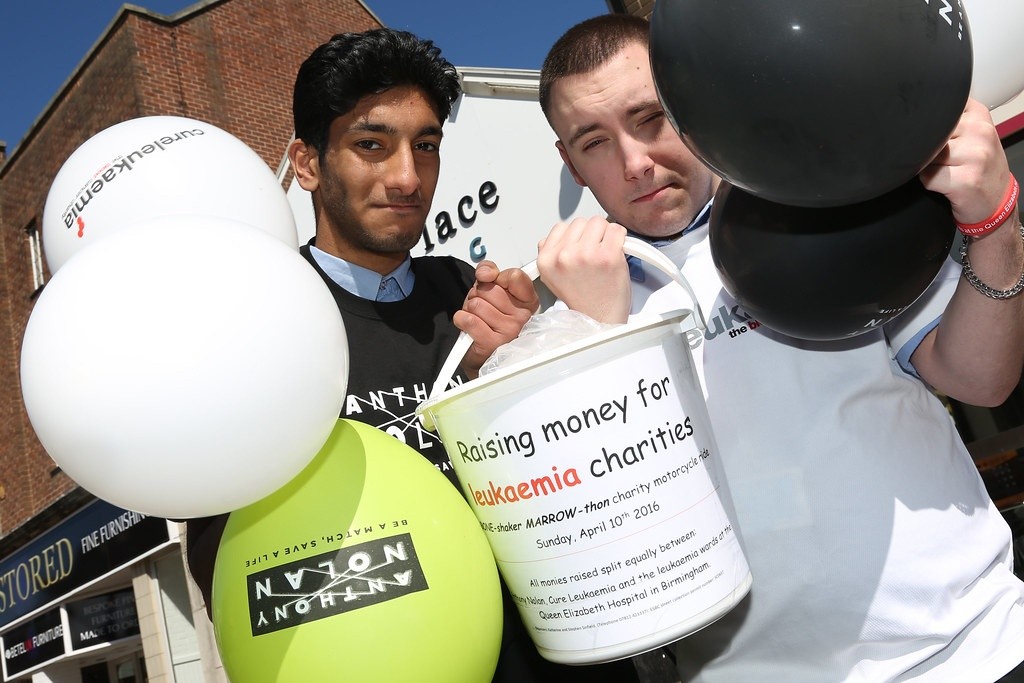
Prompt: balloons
209,415,503,683
23,219,347,511
960,2,1024,114
707,175,956,343
647,0,970,205
42,112,300,272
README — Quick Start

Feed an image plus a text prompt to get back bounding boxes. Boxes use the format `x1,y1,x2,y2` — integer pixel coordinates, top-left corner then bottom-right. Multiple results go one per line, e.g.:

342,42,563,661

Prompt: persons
514,13,1024,683
187,31,541,683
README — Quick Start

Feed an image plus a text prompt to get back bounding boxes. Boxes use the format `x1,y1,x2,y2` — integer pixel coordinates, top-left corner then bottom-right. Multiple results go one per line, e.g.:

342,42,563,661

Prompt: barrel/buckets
417,235,754,668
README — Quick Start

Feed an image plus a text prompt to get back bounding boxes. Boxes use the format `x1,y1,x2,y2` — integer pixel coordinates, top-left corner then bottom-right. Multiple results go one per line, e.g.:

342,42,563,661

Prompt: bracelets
960,233,1023,299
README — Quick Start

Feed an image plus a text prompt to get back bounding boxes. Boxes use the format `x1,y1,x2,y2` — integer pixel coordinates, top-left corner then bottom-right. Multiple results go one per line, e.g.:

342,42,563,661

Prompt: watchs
957,176,1019,236
